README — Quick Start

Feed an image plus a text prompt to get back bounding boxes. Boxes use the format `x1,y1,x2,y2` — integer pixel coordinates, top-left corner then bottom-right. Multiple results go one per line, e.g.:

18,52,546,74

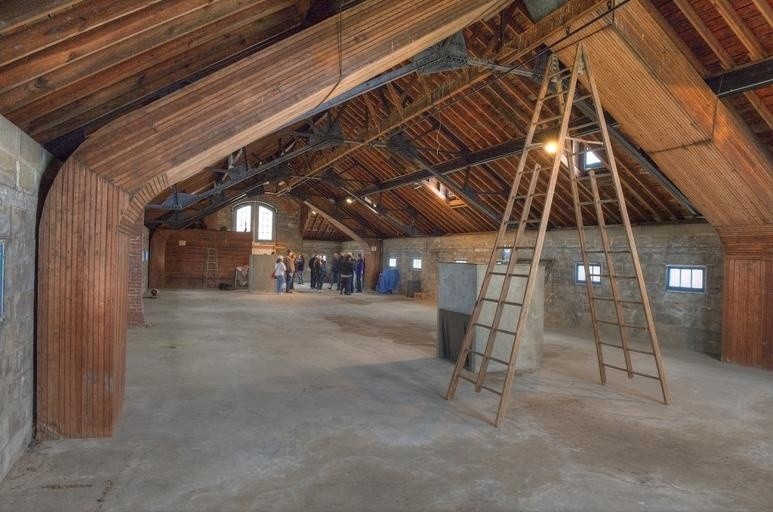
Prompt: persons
296,254,305,285
272,255,285,295
289,252,296,290
307,251,365,296
283,248,295,293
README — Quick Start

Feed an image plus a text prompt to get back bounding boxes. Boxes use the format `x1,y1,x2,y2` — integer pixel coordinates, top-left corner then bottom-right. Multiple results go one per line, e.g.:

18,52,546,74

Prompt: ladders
204,248,219,289
445,42,671,432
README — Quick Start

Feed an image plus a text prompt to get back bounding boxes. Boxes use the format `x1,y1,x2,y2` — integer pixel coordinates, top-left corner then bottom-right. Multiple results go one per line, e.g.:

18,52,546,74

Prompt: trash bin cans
406,280,420,297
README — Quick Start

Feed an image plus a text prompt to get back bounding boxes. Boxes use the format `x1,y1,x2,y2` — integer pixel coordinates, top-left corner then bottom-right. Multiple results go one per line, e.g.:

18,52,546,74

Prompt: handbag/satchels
271,272,275,279
